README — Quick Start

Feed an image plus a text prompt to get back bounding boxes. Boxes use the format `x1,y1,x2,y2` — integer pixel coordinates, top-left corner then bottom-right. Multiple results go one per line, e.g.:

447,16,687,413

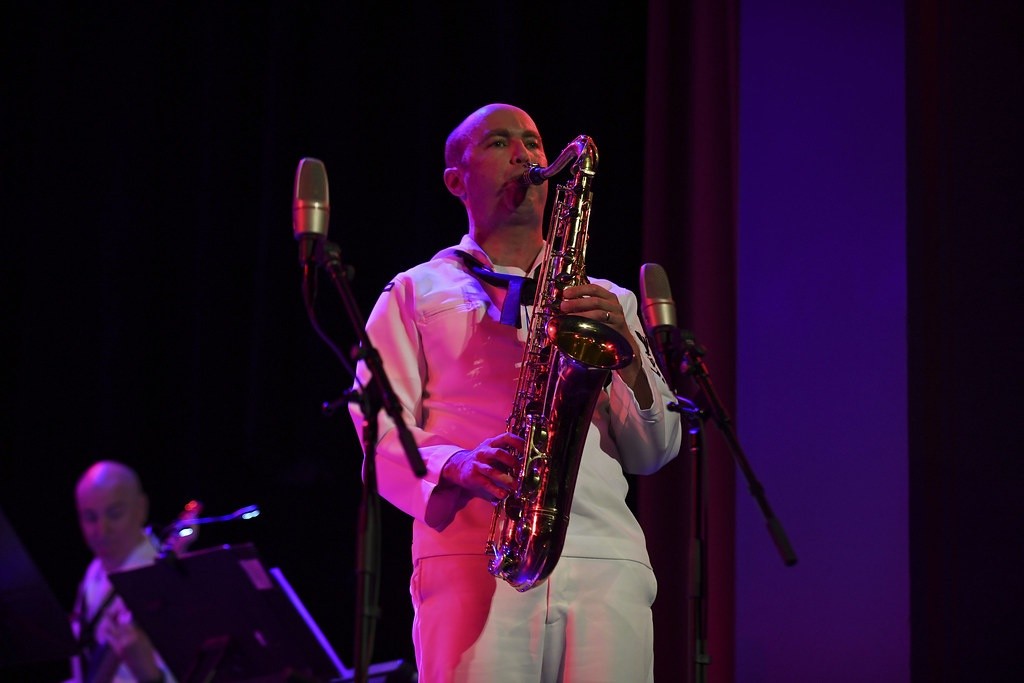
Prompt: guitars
86,497,204,683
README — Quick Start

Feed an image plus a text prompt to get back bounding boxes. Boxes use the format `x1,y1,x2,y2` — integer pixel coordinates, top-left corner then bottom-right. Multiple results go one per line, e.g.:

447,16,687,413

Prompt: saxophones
485,134,636,592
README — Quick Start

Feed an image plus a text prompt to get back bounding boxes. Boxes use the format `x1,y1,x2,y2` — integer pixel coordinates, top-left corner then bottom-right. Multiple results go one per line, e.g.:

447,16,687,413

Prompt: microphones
293,158,330,267
640,263,678,391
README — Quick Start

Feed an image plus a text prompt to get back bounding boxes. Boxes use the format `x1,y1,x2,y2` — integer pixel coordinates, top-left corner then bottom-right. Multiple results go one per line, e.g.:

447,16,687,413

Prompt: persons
65,462,175,683
347,103,682,683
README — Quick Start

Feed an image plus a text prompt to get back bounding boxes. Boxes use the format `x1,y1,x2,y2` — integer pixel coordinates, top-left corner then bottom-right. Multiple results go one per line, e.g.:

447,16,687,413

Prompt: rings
605,310,610,323
